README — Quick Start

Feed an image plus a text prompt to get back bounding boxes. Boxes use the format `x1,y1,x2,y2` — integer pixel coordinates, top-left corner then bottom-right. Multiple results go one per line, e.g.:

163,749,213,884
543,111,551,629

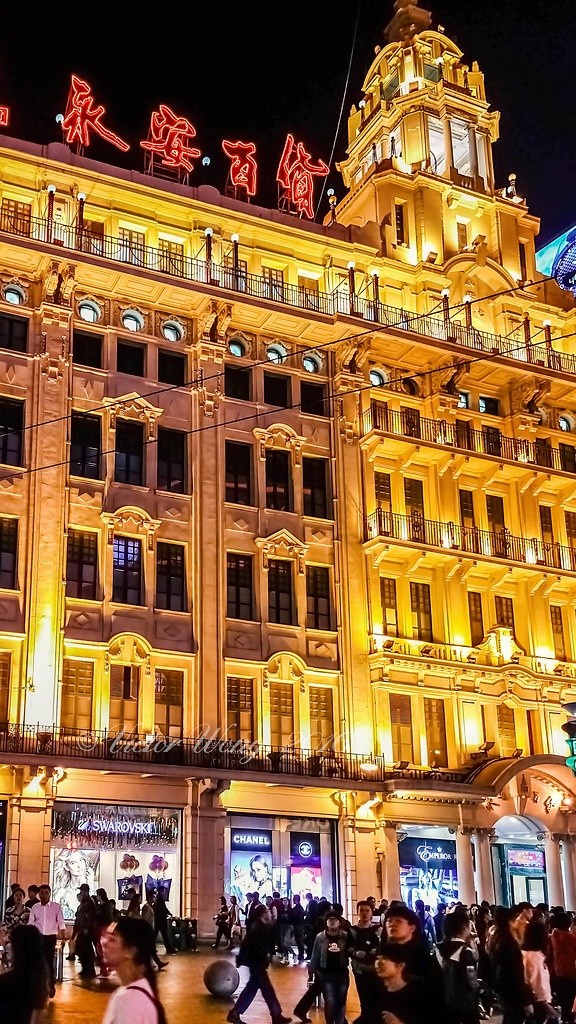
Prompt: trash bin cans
167,917,198,950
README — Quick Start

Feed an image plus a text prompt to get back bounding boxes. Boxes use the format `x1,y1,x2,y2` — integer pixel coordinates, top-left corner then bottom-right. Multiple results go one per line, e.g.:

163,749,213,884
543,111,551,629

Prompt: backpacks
440,946,473,1012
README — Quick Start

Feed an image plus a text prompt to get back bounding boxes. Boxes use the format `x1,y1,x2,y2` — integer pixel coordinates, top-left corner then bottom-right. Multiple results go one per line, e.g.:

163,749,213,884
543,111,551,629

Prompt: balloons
149,855,169,871
120,854,139,870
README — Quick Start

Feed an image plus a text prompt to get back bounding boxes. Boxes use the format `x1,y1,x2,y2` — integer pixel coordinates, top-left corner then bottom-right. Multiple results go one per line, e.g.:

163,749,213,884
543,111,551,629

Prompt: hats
76,884,89,891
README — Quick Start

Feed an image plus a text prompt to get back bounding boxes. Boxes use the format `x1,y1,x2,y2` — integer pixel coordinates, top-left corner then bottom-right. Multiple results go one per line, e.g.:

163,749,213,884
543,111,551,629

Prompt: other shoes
294,1008,312,1023
212,945,218,949
97,970,108,979
49,984,56,998
275,951,312,968
102,972,113,978
272,1014,292,1024
228,945,235,950
65,954,75,960
225,945,230,950
227,1009,247,1024
78,969,96,980
158,962,169,969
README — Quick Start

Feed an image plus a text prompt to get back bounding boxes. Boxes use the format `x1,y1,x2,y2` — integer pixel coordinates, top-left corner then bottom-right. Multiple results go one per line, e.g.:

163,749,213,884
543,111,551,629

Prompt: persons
0,924,51,1024
412,869,438,909
100,917,165,1024
53,849,95,919
0,884,576,1024
234,855,272,907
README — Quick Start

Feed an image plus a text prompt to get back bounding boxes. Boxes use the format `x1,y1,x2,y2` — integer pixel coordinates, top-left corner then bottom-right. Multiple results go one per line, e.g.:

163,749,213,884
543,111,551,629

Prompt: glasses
328,918,338,921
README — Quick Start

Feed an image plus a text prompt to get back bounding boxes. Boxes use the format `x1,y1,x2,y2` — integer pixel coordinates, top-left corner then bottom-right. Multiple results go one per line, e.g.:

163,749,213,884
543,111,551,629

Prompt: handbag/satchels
215,914,228,927
230,923,241,938
78,920,99,939
